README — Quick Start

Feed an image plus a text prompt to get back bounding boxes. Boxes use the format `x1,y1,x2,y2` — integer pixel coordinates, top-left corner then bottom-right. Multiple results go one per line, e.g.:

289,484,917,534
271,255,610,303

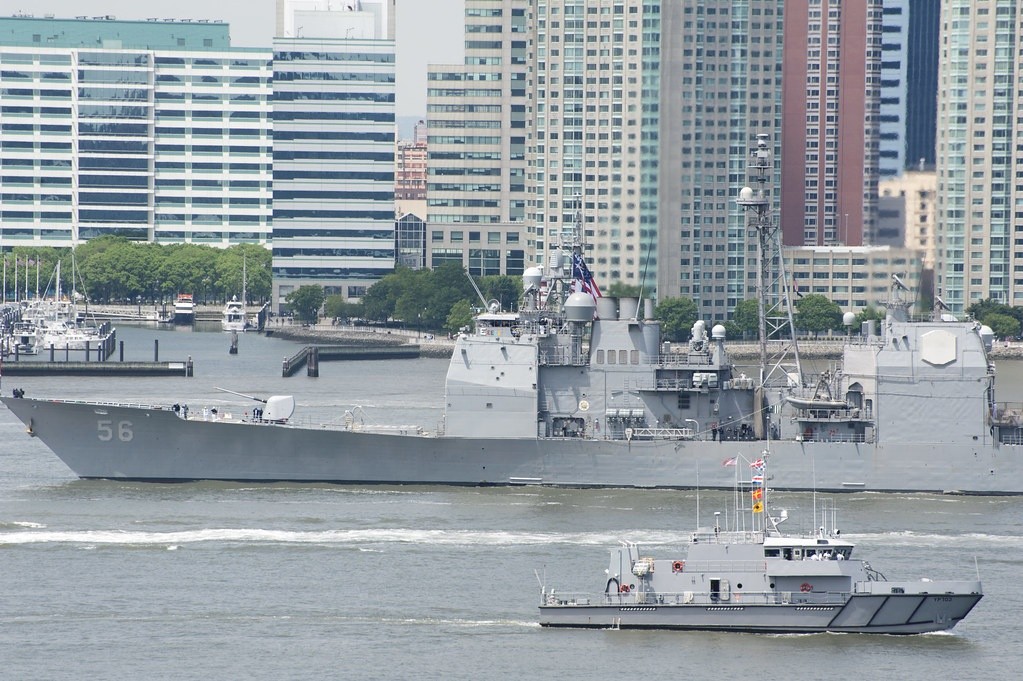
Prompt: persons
172,402,180,416
182,404,189,420
741,370,747,380
710,425,724,442
823,369,830,382
211,407,218,423
253,407,263,423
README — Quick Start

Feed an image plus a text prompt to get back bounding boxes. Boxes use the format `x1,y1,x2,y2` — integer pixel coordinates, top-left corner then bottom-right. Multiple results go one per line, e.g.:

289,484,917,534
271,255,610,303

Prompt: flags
748,459,766,515
724,455,737,467
571,250,602,305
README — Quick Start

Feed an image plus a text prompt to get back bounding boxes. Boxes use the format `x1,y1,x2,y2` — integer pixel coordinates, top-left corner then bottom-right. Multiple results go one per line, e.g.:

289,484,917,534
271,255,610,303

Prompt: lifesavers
710,424,716,429
673,561,683,571
829,429,835,436
620,584,630,592
800,582,811,592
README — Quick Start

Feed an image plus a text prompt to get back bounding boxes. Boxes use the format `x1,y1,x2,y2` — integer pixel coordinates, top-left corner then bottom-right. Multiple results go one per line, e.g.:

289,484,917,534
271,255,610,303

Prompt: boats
156,313,174,324
221,296,248,332
13,241,106,355
536,409,984,635
2,127,1023,499
